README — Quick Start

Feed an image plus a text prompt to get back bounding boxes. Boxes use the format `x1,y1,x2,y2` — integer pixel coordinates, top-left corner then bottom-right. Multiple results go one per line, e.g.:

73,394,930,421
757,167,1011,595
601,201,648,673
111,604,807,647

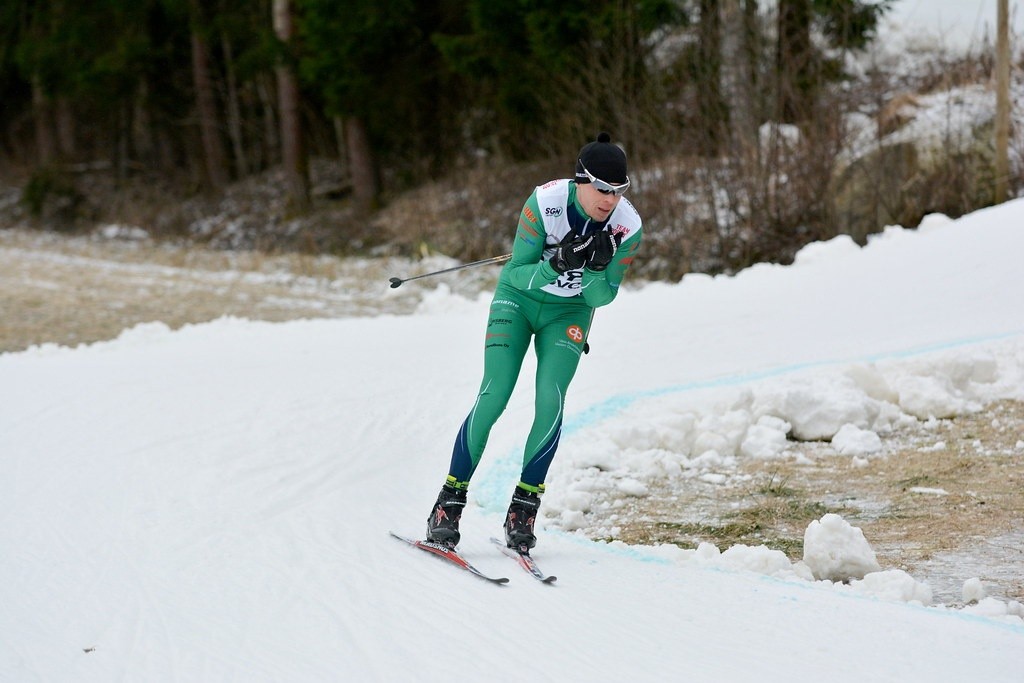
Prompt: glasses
579,159,630,197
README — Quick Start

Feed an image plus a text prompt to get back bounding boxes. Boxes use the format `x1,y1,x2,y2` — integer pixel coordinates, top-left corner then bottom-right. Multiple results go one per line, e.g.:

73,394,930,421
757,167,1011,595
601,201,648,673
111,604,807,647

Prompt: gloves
549,225,596,276
580,224,624,270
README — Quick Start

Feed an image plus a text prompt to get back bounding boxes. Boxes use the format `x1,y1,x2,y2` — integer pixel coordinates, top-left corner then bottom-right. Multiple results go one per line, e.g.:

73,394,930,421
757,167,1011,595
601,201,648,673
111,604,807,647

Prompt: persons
426,140,643,550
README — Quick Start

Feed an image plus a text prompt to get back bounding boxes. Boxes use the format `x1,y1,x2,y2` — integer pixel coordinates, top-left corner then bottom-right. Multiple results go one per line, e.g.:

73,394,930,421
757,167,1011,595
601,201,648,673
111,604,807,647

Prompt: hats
574,133,627,183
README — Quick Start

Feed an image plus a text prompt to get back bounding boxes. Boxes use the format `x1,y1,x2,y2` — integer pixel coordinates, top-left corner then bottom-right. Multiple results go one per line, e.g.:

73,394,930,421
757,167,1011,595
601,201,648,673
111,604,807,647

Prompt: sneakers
503,491,541,548
426,486,468,549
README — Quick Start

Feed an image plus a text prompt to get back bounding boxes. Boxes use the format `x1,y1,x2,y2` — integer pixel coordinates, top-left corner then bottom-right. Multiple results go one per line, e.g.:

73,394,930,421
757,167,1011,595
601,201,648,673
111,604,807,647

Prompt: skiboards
387,529,558,587
